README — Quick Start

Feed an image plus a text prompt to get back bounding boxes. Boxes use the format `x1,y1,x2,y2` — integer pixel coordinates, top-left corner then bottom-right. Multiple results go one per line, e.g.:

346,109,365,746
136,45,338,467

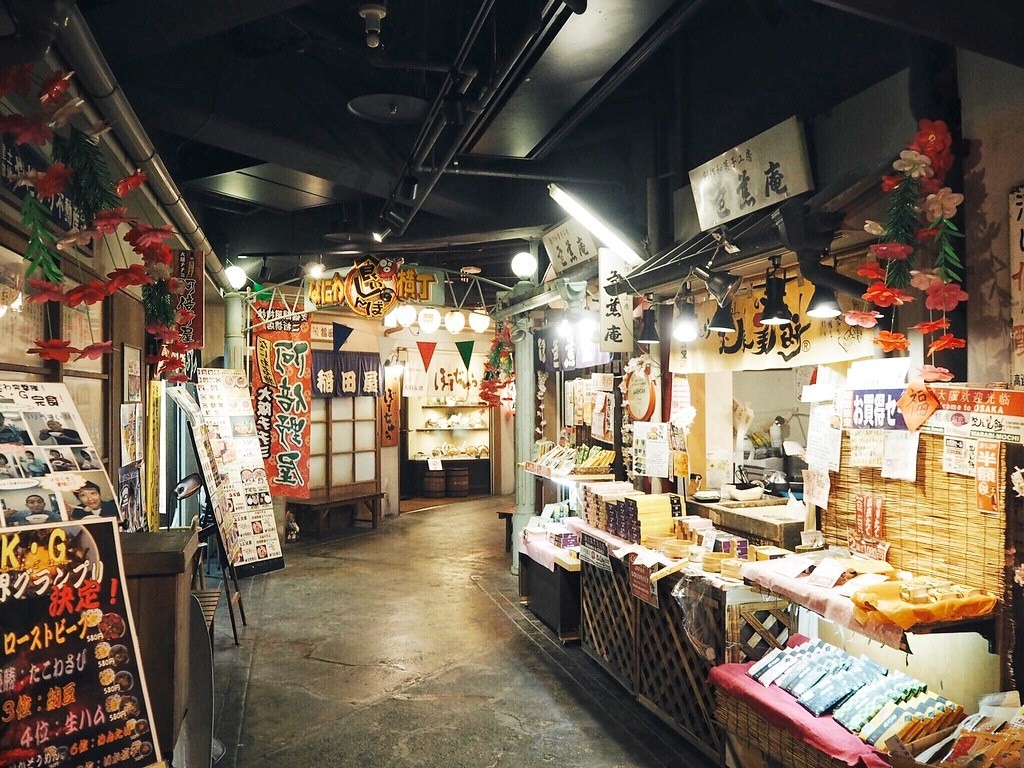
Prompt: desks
118,531,200,758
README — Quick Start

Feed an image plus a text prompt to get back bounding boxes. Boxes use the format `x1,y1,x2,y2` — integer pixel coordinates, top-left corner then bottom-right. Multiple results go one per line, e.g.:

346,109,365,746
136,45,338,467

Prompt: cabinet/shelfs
561,516,818,768
519,530,581,644
416,405,489,431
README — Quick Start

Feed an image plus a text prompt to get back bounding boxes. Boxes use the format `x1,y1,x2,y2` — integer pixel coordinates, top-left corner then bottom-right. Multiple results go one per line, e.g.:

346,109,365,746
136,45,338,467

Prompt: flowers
535,370,549,439
621,354,652,481
1,59,202,383
842,119,969,383
479,317,515,424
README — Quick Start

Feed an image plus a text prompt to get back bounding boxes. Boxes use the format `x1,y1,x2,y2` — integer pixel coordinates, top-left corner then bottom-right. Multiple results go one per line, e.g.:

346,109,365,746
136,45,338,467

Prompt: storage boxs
698,530,748,559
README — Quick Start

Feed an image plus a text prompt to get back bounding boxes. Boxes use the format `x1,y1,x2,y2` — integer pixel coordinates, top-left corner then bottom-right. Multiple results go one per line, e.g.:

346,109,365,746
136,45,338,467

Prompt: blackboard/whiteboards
0,525,160,768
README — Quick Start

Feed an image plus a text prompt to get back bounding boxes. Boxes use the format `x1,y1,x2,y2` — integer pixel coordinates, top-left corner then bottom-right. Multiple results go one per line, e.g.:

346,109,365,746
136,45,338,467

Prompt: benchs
191,588,221,655
286,491,386,541
496,506,516,552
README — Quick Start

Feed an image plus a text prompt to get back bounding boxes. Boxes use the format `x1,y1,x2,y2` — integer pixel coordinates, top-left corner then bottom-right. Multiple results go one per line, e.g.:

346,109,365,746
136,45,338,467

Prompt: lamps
383,209,405,229
547,183,650,269
224,266,247,292
258,257,272,281
402,161,419,201
511,252,538,297
805,253,843,319
372,224,392,244
558,280,588,308
759,255,792,325
449,63,478,99
706,271,736,333
501,318,526,345
691,225,742,308
670,282,700,342
491,289,562,322
445,102,464,129
396,304,490,334
636,292,660,344
293,255,305,278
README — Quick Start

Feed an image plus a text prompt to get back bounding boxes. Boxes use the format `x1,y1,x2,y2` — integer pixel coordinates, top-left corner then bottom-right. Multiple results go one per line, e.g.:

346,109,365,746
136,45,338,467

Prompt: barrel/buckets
422,467,469,497
